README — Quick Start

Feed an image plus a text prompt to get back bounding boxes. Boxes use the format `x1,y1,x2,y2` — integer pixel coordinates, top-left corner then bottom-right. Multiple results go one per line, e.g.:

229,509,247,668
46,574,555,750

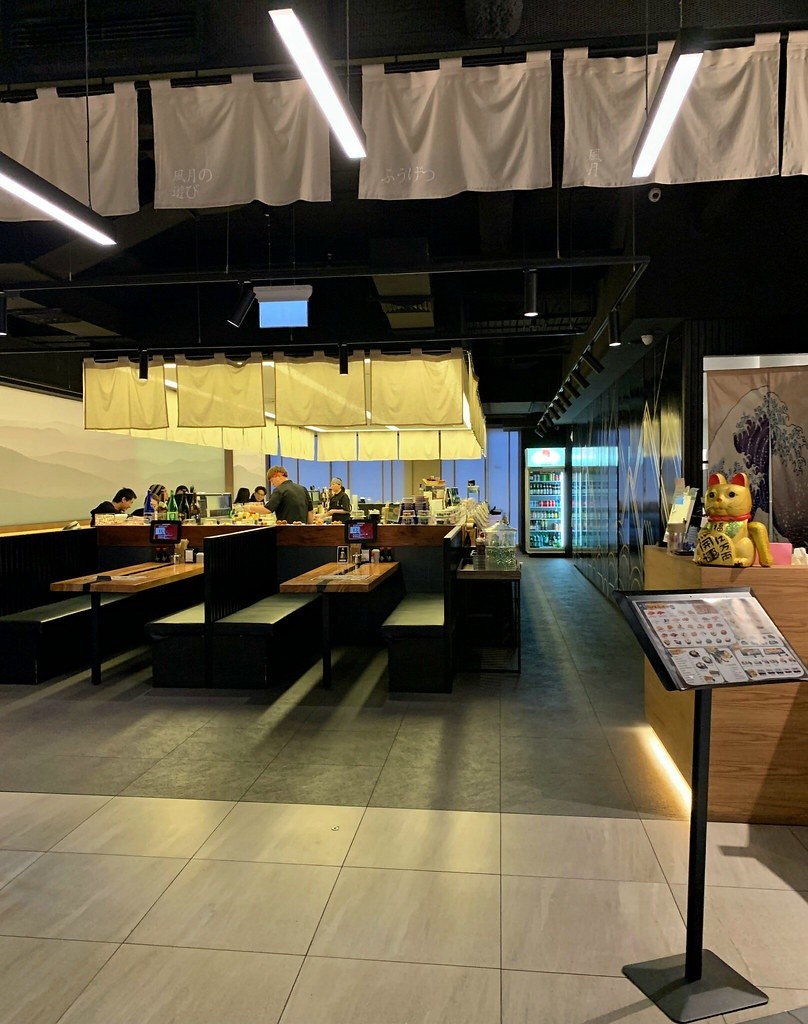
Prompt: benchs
381,524,464,692
144,602,205,689
0,529,133,686
203,526,322,693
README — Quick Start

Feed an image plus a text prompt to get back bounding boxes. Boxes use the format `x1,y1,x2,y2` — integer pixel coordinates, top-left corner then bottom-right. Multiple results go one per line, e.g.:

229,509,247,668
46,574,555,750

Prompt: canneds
532,473,561,481
539,500,560,506
531,511,561,519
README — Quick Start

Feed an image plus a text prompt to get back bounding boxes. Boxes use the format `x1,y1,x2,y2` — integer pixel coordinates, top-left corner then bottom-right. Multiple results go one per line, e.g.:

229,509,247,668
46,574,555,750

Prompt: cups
345,489,358,510
182,516,233,525
115,514,128,523
230,501,269,525
95,513,115,525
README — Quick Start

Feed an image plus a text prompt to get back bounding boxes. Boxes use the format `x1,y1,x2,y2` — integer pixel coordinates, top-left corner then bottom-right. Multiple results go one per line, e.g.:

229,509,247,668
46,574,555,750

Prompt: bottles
668,523,685,555
337,546,392,563
529,470,562,548
155,546,204,564
464,530,486,556
320,487,334,508
154,485,201,524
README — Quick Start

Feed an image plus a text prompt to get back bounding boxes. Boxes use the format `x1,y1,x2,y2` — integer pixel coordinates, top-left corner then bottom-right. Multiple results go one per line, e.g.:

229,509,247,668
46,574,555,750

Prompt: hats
148,484,165,498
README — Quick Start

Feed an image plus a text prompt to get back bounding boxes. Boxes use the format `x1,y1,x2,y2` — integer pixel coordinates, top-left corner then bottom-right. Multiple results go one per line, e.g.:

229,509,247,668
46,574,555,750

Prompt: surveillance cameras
641,335,653,345
648,187,661,202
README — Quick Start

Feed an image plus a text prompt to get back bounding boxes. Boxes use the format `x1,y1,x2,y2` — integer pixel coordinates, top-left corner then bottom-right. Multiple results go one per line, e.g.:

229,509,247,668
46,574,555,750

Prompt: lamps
640,332,656,345
0,12,118,249
338,339,348,376
266,1,368,160
521,265,541,318
608,306,621,348
227,280,258,327
533,340,605,439
632,0,706,179
137,345,148,380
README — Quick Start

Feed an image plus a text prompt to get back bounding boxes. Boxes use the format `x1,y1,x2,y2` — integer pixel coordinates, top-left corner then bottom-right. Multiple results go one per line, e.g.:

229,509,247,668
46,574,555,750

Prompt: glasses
257,492,265,496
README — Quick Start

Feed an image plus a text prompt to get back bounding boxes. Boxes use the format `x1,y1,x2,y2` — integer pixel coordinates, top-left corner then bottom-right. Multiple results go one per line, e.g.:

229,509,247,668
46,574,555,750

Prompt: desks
279,562,399,690
50,561,205,687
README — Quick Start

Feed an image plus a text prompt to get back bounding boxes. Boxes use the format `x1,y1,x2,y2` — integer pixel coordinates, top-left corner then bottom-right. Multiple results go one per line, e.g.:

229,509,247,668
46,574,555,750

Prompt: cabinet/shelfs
458,559,521,677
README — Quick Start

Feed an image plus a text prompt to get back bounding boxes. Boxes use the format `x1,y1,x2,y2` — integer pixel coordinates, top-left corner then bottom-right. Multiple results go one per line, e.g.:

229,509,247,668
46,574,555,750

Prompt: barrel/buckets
485,514,517,571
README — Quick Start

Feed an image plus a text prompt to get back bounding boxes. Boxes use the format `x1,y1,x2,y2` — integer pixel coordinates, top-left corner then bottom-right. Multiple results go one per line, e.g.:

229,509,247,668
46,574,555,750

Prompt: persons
90,488,137,527
242,466,313,524
340,486,345,493
234,486,267,505
130,484,200,521
327,478,350,539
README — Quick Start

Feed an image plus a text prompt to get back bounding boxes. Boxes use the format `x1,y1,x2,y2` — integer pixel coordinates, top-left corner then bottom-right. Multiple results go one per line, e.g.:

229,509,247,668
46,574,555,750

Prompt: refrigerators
524,448,566,557
572,447,619,557
